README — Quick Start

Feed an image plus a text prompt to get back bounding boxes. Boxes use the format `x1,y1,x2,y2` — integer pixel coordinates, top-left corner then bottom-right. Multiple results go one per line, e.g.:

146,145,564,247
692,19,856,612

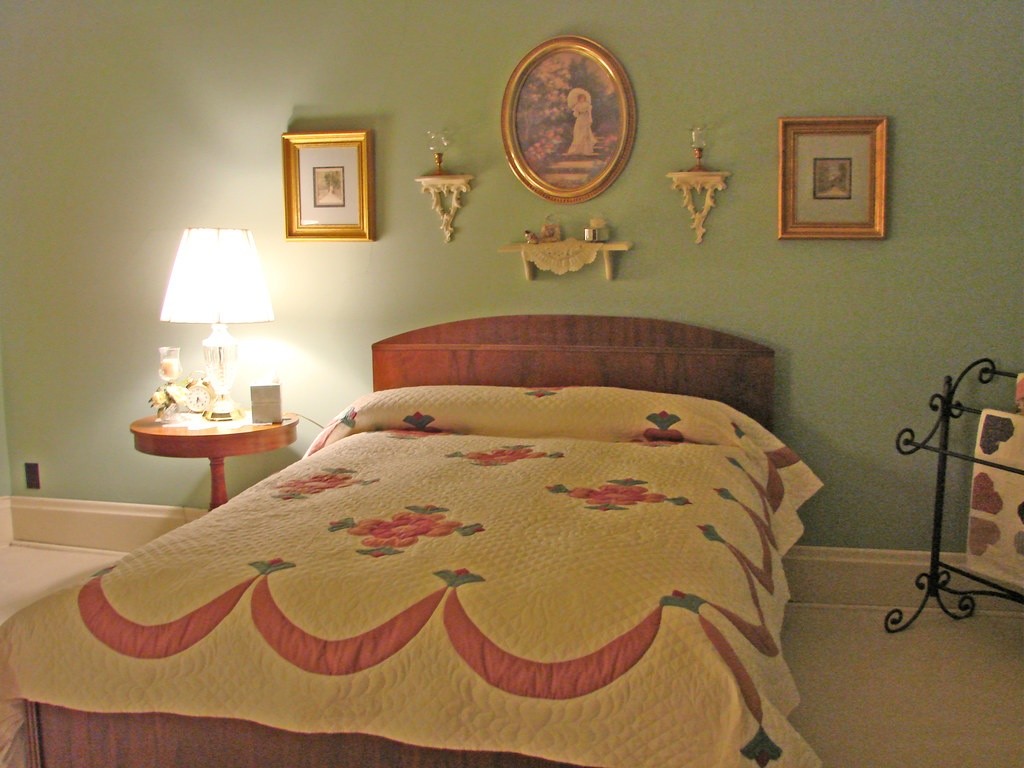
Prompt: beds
0,313,824,768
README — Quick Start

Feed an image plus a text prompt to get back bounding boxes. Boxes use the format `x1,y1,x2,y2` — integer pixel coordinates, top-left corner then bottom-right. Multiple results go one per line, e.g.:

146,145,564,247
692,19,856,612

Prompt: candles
156,347,183,384
582,216,612,243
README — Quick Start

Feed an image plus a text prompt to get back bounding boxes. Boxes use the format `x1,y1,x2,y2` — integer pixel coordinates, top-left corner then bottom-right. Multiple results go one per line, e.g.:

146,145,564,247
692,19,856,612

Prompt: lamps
160,228,273,422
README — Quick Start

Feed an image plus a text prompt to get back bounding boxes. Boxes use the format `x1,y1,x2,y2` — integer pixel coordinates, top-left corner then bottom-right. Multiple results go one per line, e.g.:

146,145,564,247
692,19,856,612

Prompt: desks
131,412,298,513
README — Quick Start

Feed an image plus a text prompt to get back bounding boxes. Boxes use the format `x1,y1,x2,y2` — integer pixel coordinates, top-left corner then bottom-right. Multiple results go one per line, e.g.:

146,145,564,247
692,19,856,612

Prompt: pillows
302,385,736,446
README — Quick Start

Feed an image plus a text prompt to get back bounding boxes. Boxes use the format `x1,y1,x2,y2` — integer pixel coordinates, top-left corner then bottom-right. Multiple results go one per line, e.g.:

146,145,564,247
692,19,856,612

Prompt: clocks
184,370,216,415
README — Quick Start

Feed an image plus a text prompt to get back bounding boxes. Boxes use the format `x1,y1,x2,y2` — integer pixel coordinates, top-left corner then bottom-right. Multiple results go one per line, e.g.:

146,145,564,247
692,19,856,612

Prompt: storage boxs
250,402,283,424
250,381,283,403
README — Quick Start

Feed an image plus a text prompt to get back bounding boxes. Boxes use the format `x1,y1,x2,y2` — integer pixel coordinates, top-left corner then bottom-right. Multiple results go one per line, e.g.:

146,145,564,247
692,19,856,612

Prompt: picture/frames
499,35,636,203
777,116,886,240
280,129,377,243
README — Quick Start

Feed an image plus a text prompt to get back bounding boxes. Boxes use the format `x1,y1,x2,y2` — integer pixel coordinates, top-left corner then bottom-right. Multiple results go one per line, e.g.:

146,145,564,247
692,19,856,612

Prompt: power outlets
25,462,40,489
